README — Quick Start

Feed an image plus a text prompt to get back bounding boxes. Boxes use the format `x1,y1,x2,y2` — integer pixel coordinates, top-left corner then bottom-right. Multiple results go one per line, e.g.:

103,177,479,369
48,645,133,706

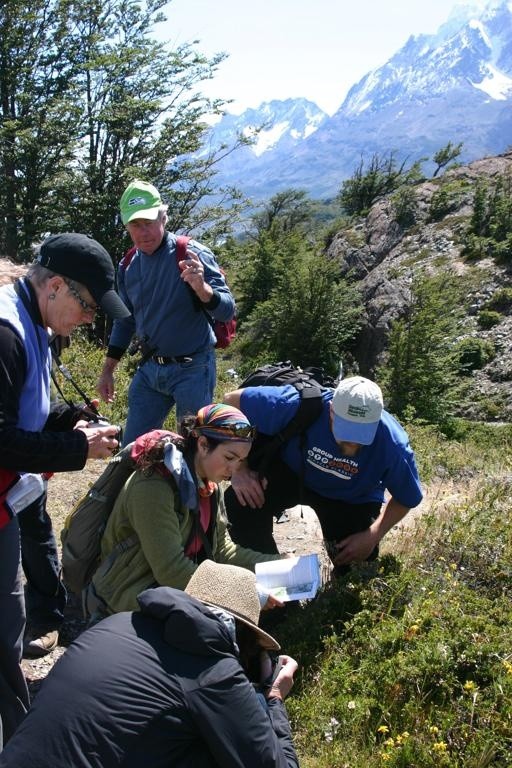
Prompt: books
250,553,321,603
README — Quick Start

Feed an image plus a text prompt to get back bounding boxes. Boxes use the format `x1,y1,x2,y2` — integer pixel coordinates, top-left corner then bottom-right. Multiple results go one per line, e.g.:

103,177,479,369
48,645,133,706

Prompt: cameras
91,418,123,456
240,643,284,689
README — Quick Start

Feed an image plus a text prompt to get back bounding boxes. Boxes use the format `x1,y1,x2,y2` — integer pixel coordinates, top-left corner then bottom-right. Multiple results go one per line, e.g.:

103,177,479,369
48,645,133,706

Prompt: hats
35,233,132,320
118,181,162,225
184,558,281,651
333,375,384,446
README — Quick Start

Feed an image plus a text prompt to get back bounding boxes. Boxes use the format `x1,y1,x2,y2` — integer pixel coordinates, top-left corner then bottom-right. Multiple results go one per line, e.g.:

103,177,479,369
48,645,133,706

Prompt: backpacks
60,441,185,593
123,235,236,348
238,360,335,473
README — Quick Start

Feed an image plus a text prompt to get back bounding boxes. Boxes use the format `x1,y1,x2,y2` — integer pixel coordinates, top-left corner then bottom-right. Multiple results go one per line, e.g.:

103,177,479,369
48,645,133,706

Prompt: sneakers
23,620,64,660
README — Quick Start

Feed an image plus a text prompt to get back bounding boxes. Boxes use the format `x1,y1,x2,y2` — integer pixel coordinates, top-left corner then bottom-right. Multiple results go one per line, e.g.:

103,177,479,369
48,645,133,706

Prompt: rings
191,266,197,274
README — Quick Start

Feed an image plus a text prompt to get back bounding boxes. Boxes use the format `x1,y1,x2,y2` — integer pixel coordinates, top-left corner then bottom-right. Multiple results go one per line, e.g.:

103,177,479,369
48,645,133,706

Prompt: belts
154,351,203,367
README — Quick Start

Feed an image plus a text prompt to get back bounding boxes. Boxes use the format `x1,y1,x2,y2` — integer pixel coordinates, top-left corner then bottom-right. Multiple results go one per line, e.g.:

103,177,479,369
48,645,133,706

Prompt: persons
94,179,236,453
81,403,297,627
222,376,423,575
2,234,131,745
2,559,302,767
19,470,66,655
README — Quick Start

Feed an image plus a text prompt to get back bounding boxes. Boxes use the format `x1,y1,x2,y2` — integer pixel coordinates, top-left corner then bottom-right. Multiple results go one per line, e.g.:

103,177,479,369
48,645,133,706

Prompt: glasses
47,275,98,318
231,423,258,440
